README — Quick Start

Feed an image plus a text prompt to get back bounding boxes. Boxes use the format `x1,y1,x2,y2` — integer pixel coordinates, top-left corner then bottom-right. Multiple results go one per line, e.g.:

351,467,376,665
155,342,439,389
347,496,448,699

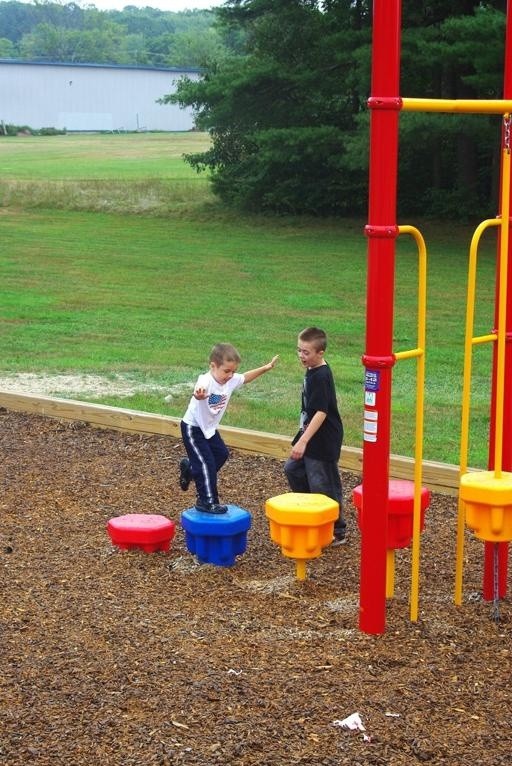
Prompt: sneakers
179,459,193,492
328,532,347,547
195,497,228,514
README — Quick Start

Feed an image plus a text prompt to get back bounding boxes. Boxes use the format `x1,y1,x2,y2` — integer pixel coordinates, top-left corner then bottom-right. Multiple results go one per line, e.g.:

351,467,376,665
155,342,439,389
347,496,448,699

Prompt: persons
283,327,346,545
178,342,282,514
